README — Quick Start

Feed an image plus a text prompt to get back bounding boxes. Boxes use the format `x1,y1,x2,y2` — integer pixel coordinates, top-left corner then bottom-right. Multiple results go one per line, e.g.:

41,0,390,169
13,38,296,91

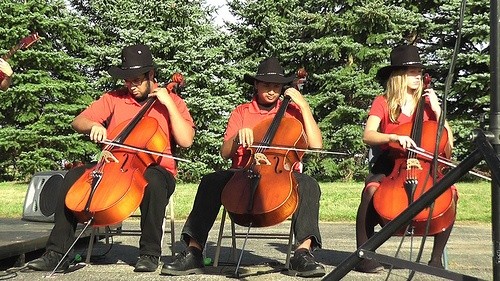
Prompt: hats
108,44,167,79
376,45,426,82
244,57,295,86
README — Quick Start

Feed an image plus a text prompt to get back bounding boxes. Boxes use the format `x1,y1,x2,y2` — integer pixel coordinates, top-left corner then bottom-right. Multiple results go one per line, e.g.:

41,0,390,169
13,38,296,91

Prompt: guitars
0,29,38,81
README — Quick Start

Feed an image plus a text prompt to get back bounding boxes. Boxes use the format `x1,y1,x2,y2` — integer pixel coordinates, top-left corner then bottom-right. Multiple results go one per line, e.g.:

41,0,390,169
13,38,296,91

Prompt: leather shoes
27,250,69,270
134,254,159,272
288,250,325,277
160,251,206,276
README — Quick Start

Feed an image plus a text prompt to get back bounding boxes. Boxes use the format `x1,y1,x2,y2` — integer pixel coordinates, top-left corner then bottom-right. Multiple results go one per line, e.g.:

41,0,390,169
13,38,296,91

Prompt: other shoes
354,258,385,273
428,261,445,270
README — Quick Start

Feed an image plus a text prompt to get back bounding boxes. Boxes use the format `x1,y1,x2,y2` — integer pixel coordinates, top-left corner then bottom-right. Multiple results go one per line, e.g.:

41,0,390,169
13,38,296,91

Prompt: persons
357,45,462,274
27,44,195,272
0,57,14,91
160,58,325,277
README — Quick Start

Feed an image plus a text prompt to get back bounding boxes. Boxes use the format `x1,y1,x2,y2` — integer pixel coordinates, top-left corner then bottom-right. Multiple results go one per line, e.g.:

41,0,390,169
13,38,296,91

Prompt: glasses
122,75,146,87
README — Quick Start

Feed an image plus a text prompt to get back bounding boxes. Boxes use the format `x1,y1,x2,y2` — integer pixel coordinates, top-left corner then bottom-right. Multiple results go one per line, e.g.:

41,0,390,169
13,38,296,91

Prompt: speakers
20,168,68,223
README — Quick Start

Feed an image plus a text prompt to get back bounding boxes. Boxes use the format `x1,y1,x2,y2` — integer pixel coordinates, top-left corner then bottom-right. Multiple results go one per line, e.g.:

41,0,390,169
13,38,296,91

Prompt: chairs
363,147,459,273
212,156,305,271
85,182,177,267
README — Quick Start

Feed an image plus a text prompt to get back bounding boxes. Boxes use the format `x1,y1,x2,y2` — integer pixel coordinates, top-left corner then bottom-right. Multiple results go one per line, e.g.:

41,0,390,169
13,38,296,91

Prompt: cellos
373,71,458,281
220,65,310,275
45,73,186,281
